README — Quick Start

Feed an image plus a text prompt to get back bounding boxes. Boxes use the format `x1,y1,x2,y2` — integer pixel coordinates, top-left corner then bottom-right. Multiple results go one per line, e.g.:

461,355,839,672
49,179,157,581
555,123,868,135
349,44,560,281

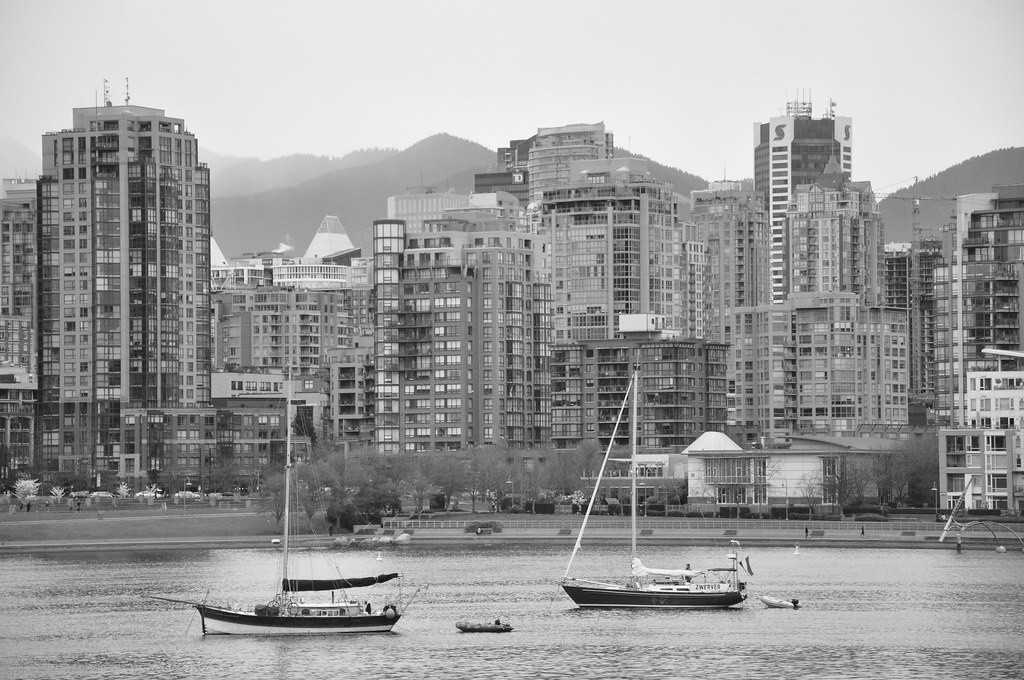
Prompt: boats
760,595,802,611
454,620,514,633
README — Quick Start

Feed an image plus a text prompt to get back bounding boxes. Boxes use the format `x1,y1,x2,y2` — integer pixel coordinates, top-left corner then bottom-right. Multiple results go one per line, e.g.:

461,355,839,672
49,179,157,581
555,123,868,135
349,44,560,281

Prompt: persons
27,503,31,512
805,526,808,538
573,503,581,516
364,601,371,614
684,564,692,582
936,513,945,522
20,503,23,510
46,502,50,512
329,524,332,536
861,526,864,536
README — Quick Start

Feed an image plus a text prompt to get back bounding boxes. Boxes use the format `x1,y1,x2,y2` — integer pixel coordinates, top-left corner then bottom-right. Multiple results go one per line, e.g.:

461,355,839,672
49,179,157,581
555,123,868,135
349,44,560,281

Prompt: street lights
638,476,647,518
506,474,514,509
182,476,193,517
931,481,940,522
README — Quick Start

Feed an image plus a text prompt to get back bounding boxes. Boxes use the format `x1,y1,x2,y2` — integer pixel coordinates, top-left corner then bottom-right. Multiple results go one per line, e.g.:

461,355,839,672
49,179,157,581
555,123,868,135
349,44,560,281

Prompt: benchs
382,530,394,535
401,530,415,535
479,528,492,535
901,532,916,536
723,530,737,535
558,530,571,535
810,531,825,538
636,530,653,536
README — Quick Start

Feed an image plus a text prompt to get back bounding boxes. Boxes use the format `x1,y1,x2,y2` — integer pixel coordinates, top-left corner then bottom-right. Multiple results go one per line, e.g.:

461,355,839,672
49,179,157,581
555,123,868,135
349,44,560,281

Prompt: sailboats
552,368,757,612
148,348,428,636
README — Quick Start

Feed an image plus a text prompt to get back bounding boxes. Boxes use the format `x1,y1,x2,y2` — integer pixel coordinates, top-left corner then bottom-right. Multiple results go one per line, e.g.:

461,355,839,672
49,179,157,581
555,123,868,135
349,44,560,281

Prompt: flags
740,558,753,576
